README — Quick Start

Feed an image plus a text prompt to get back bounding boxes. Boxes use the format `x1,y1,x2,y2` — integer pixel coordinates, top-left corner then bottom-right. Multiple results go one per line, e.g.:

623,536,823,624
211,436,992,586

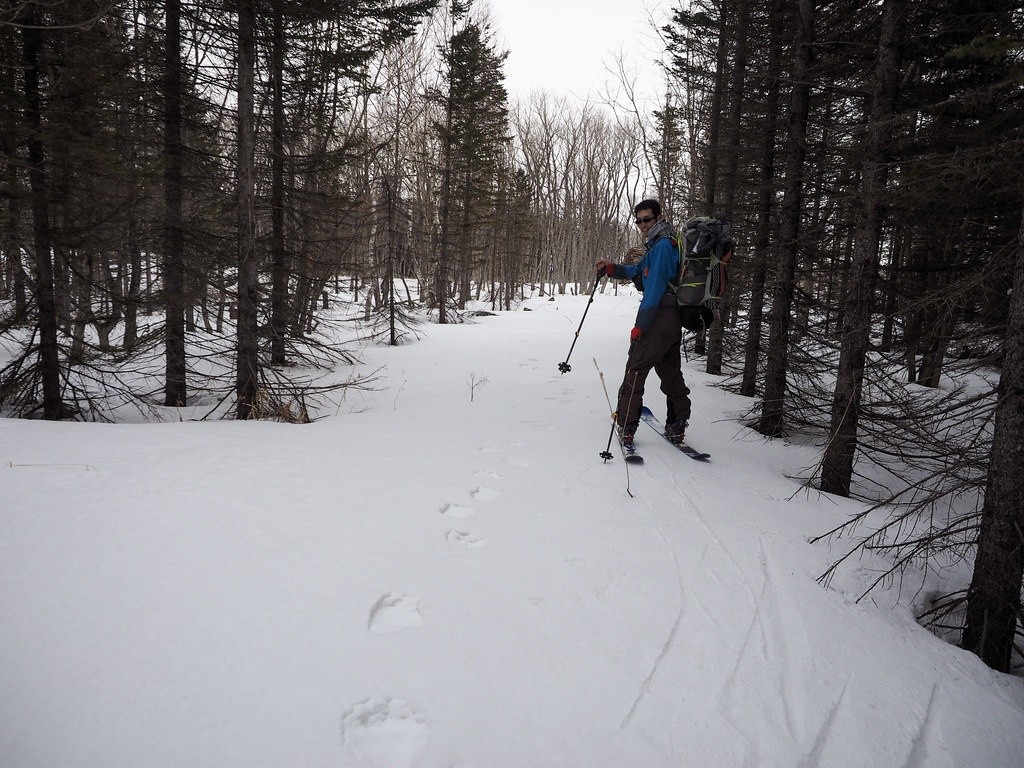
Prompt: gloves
596,260,614,275
631,327,642,343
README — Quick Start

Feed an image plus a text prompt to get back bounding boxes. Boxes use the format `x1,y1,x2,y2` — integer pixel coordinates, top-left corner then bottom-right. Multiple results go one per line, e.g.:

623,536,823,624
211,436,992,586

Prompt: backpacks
650,217,734,332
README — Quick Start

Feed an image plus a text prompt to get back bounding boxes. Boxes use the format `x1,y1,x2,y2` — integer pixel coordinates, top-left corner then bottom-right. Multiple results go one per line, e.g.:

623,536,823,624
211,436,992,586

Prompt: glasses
635,217,655,224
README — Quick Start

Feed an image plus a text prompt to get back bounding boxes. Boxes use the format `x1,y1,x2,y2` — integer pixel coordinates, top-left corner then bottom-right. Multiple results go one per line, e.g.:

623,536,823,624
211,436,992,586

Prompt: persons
596,199,691,443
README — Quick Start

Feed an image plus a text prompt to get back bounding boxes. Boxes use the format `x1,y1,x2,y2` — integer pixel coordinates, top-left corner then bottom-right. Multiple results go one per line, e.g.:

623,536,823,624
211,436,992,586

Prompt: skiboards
609,403,715,464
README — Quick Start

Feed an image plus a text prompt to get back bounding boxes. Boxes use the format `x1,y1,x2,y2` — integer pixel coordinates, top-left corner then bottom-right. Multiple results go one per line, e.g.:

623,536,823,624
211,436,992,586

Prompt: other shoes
664,423,688,442
616,424,639,443
